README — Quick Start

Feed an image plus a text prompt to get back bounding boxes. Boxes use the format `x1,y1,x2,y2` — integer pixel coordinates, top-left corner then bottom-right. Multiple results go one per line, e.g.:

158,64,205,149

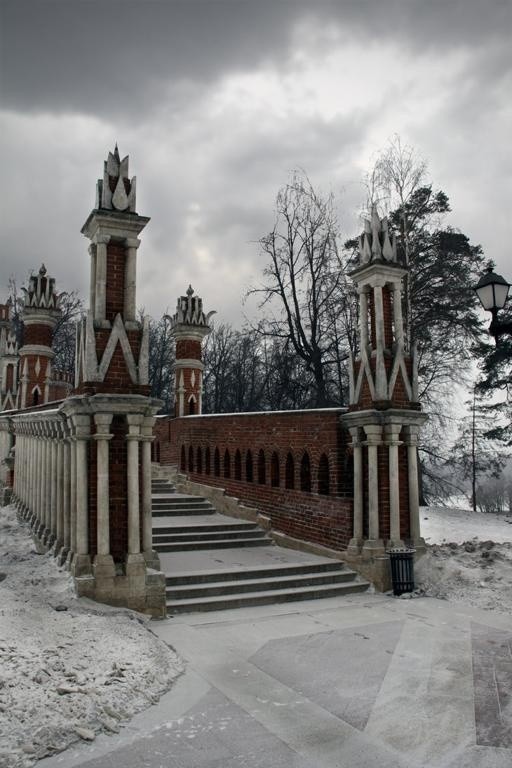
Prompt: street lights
472,260,512,345
